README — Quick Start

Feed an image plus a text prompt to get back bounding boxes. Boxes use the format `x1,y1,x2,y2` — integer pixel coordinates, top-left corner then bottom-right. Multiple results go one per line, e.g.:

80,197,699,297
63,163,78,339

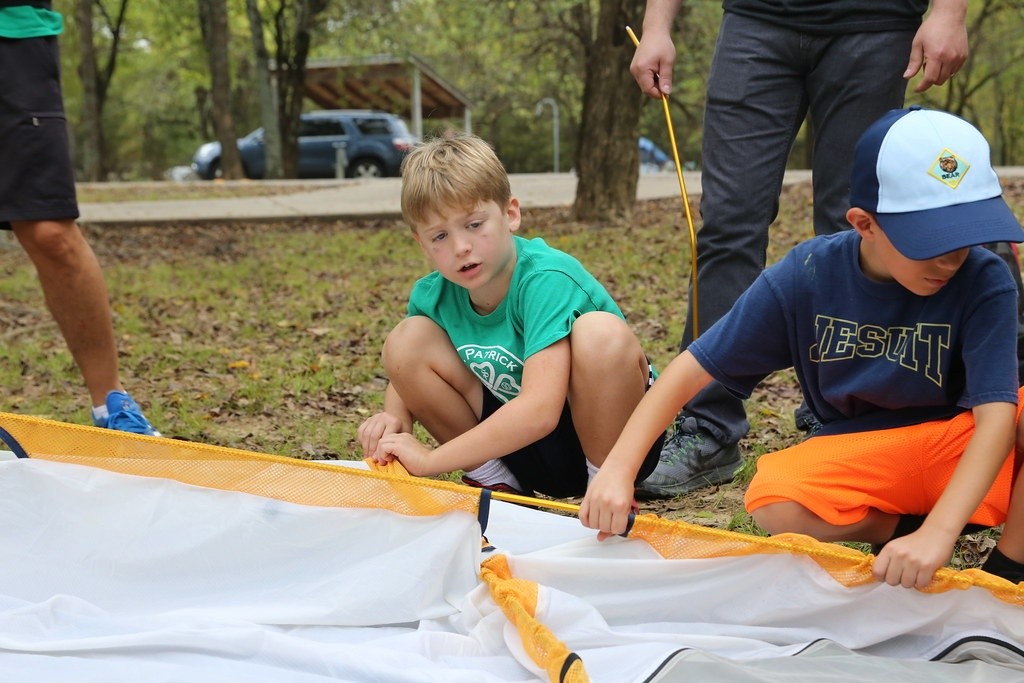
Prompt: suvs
191,109,416,180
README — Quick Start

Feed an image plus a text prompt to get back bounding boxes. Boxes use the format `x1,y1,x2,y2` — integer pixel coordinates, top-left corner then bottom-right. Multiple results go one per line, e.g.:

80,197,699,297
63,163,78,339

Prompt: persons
357,132,667,509
0,0,161,437
630,0,976,492
578,105,1024,589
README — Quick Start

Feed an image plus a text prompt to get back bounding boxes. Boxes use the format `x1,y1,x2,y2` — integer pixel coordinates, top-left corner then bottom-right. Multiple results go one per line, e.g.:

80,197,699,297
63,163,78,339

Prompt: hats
848,104,1024,260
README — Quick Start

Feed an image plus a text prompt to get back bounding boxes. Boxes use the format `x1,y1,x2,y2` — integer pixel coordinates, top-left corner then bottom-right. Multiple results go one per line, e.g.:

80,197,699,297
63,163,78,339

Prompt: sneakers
636,413,748,499
90,389,162,439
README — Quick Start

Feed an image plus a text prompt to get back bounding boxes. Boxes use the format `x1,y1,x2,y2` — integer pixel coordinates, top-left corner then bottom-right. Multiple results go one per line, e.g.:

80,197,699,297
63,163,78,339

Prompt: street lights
535,97,559,172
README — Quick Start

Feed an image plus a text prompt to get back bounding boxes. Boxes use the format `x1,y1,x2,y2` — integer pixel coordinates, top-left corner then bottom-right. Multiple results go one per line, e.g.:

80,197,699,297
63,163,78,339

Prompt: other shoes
461,474,534,499
